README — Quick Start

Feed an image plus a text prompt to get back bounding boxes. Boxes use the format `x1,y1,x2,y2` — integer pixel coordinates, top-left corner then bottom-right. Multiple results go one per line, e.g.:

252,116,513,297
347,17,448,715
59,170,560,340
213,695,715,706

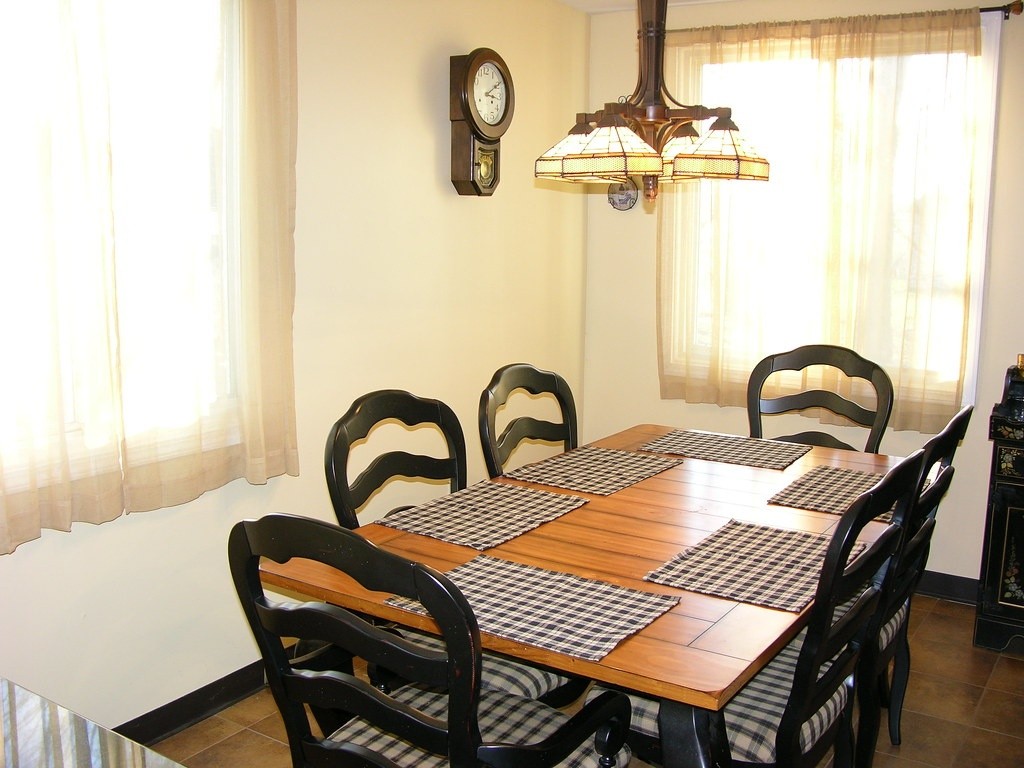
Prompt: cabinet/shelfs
973,354,1024,655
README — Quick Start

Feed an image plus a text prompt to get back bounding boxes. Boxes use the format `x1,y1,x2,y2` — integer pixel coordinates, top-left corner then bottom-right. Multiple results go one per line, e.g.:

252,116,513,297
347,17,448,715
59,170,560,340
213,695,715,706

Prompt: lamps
535,0,769,202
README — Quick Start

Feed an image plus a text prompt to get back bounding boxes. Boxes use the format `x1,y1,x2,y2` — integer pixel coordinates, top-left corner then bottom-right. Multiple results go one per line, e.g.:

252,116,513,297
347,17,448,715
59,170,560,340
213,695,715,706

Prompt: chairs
747,345,893,456
228,513,632,768
478,363,577,479
584,404,976,768
324,389,591,712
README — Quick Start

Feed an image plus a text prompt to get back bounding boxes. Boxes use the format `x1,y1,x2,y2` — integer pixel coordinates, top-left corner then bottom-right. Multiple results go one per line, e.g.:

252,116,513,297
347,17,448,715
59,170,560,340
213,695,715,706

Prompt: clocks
459,47,515,141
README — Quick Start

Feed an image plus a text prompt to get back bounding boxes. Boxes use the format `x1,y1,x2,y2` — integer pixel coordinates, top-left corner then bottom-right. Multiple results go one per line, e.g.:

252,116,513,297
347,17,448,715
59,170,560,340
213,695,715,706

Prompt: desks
256,423,942,768
